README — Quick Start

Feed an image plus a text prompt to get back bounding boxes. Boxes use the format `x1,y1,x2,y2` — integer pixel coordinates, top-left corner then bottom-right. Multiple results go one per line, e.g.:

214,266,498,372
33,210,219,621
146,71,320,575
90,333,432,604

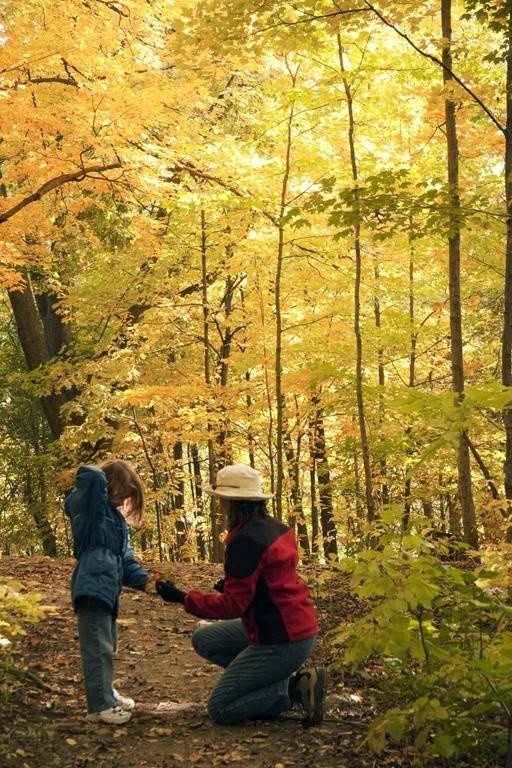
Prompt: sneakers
292,667,325,728
88,686,135,726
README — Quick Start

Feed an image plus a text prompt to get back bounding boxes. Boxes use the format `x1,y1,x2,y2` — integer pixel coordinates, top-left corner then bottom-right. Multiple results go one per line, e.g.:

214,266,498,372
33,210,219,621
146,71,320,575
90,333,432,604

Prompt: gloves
154,578,187,605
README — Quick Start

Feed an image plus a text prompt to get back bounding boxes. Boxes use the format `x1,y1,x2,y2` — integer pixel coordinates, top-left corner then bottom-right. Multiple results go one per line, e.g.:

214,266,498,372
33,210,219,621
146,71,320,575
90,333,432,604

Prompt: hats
204,464,276,502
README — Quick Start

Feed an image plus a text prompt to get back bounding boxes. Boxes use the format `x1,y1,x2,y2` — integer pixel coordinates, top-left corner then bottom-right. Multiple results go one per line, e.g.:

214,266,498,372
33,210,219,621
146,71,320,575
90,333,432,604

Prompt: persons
155,464,327,726
63,459,163,724
